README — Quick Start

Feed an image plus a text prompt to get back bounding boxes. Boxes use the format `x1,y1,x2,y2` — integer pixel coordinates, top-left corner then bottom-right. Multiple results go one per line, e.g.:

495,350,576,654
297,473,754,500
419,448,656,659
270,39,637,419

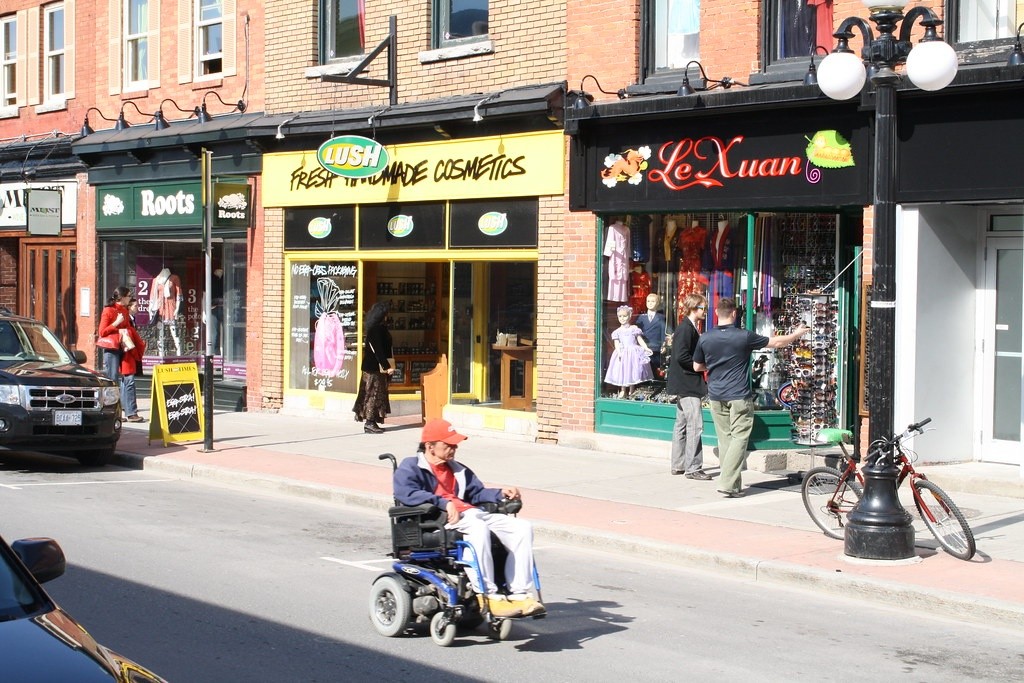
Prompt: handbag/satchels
98,332,121,352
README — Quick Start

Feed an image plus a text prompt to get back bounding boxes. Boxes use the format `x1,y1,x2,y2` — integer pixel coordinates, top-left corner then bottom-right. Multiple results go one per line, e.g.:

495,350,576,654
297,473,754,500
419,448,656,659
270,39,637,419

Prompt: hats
420,418,467,444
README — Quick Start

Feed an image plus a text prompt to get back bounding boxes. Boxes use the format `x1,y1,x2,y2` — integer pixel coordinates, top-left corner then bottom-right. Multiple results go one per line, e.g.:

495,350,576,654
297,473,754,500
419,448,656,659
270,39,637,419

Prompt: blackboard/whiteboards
149,362,204,442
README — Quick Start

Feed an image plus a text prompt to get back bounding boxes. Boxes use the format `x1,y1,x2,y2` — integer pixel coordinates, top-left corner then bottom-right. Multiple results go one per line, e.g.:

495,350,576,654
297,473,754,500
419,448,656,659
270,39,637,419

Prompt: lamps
274,114,299,139
677,61,747,97
803,44,829,86
1006,22,1024,67
572,73,627,109
368,105,388,124
473,91,496,122
81,91,245,137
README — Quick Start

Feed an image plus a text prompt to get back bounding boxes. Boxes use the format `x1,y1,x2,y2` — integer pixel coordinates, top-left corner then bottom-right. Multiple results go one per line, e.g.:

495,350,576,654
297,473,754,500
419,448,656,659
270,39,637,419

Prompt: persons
677,219,707,333
651,218,684,333
603,305,654,398
635,292,666,381
351,303,395,434
201,268,224,354
630,213,652,262
98,286,144,422
147,268,185,357
393,418,546,617
666,292,711,480
691,295,812,498
127,298,139,331
701,218,737,275
605,220,632,303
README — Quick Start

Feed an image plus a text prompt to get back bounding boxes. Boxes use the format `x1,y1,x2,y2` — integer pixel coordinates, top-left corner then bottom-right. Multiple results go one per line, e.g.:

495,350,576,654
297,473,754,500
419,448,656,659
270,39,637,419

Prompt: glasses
695,305,707,311
773,212,838,443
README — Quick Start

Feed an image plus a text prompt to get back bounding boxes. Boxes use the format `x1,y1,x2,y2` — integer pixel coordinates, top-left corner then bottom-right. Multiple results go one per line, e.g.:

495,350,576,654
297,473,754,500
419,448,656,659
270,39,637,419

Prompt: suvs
1,305,122,467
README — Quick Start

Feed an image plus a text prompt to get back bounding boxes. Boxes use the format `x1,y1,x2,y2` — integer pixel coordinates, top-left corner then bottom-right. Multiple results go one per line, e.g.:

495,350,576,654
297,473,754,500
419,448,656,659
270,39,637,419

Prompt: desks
491,342,537,410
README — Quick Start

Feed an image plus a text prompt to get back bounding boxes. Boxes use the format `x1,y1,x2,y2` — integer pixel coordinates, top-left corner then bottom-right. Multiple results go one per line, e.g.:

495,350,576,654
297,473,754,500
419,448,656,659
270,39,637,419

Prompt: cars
1,535,167,683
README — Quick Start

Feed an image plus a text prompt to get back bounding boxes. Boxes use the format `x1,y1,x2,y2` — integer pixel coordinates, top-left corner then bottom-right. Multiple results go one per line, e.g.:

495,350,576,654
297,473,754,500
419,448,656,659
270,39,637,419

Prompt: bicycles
801,418,976,561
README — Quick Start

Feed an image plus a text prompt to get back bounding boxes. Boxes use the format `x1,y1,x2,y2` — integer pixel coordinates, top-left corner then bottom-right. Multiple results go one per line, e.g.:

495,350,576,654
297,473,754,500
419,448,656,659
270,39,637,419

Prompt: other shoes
510,598,546,616
686,470,714,480
121,418,127,422
722,490,746,498
477,596,522,618
364,422,385,434
128,414,144,422
672,470,684,475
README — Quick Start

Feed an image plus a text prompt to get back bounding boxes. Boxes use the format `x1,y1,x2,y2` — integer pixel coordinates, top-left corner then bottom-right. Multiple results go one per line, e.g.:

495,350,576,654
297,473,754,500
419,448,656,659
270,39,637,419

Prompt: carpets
749,469,863,495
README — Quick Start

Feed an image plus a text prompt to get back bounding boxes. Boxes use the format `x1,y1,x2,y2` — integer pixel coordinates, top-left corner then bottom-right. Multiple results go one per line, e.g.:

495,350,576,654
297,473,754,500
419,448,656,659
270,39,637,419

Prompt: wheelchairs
369,454,547,647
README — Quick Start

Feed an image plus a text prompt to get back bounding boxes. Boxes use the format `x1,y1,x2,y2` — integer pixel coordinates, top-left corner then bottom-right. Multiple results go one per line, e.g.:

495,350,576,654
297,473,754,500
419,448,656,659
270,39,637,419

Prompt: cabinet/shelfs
376,293,439,390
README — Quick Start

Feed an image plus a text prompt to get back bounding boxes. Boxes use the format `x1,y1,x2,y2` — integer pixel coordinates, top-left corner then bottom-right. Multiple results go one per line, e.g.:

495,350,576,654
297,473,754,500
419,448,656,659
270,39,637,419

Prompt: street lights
816,0,958,560
